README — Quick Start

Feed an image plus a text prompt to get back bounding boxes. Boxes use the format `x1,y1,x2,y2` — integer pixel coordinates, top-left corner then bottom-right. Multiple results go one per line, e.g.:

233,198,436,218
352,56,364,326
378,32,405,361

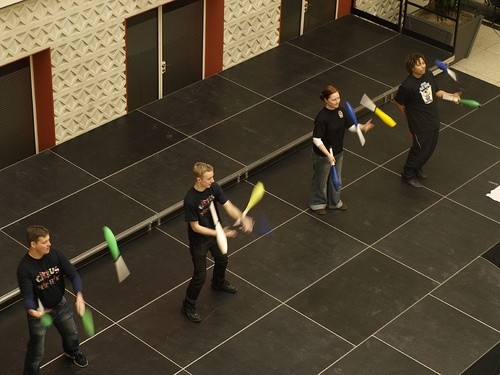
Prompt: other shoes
402,175,421,188
211,278,238,293
65,351,88,368
416,168,428,179
181,302,201,322
315,209,326,214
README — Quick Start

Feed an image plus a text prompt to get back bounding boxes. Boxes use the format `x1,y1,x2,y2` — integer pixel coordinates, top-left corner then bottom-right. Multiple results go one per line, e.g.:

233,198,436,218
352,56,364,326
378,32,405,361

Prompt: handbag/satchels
339,203,348,209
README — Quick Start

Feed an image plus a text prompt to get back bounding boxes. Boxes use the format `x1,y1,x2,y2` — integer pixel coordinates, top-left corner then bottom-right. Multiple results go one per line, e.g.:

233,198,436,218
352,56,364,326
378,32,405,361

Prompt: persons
393,52,462,189
16,225,88,375
181,162,254,322
308,86,375,215
